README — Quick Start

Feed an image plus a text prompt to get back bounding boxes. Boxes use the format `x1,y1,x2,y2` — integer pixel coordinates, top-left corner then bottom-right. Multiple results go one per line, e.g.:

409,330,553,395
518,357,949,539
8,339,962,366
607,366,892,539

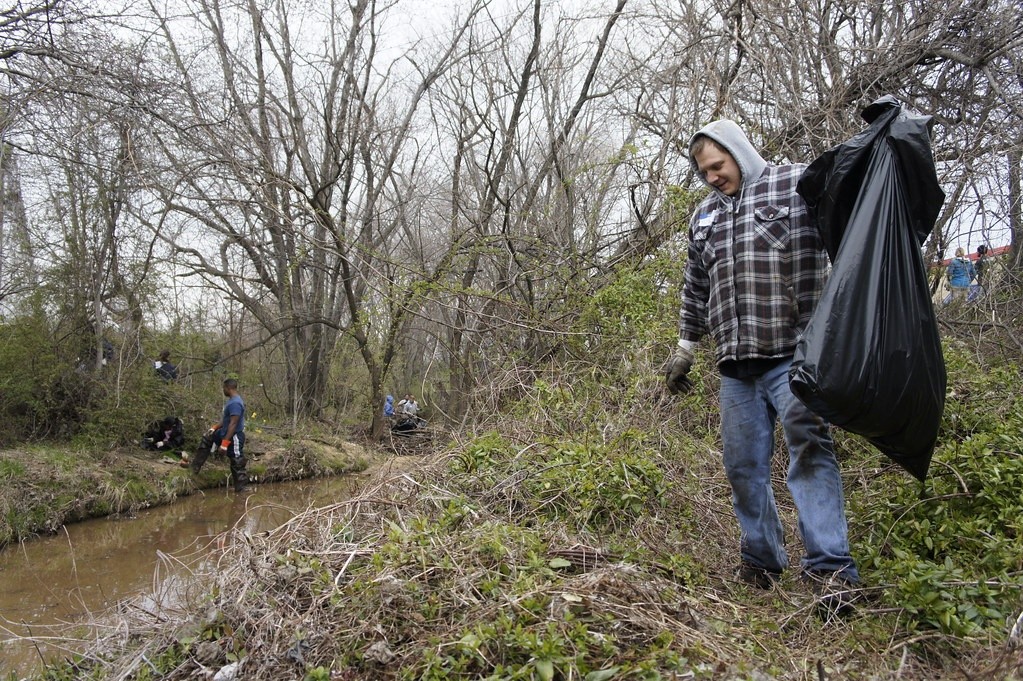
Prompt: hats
955,248,964,256
977,245,987,254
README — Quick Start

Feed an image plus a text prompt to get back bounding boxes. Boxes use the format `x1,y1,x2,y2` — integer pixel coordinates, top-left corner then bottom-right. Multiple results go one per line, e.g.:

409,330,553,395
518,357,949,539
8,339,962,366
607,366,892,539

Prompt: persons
945,248,974,300
397,394,422,423
665,119,857,614
154,351,175,378
384,396,395,416
974,245,991,286
143,417,183,449
185,378,248,491
84,326,113,363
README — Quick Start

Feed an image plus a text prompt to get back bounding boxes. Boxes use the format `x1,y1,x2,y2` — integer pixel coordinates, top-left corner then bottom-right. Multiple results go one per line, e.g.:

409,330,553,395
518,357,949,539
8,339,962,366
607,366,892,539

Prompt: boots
181,434,215,475
230,456,250,492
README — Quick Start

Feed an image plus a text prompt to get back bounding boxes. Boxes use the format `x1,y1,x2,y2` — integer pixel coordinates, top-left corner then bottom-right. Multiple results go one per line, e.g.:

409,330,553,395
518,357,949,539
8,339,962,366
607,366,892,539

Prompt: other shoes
734,562,775,589
815,571,852,619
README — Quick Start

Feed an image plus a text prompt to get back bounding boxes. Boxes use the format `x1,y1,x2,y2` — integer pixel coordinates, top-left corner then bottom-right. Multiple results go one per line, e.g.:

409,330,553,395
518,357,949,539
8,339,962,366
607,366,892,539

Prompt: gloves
665,346,695,394
944,281,950,291
215,440,231,463
203,424,219,437
